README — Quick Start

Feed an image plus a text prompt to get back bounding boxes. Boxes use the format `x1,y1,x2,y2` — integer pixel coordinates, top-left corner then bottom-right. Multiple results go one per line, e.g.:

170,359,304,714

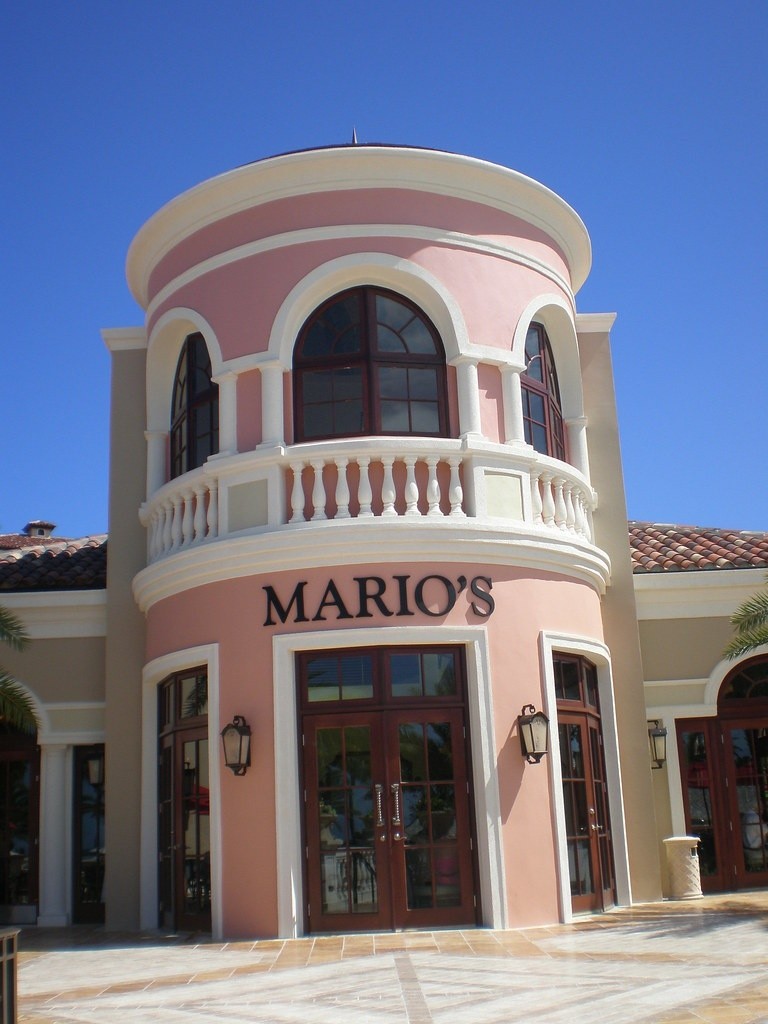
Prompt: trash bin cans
663,836,705,900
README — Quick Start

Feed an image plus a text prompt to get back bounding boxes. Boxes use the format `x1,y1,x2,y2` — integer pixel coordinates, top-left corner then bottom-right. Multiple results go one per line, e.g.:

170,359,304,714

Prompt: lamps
218,715,251,776
646,719,668,770
518,704,549,764
